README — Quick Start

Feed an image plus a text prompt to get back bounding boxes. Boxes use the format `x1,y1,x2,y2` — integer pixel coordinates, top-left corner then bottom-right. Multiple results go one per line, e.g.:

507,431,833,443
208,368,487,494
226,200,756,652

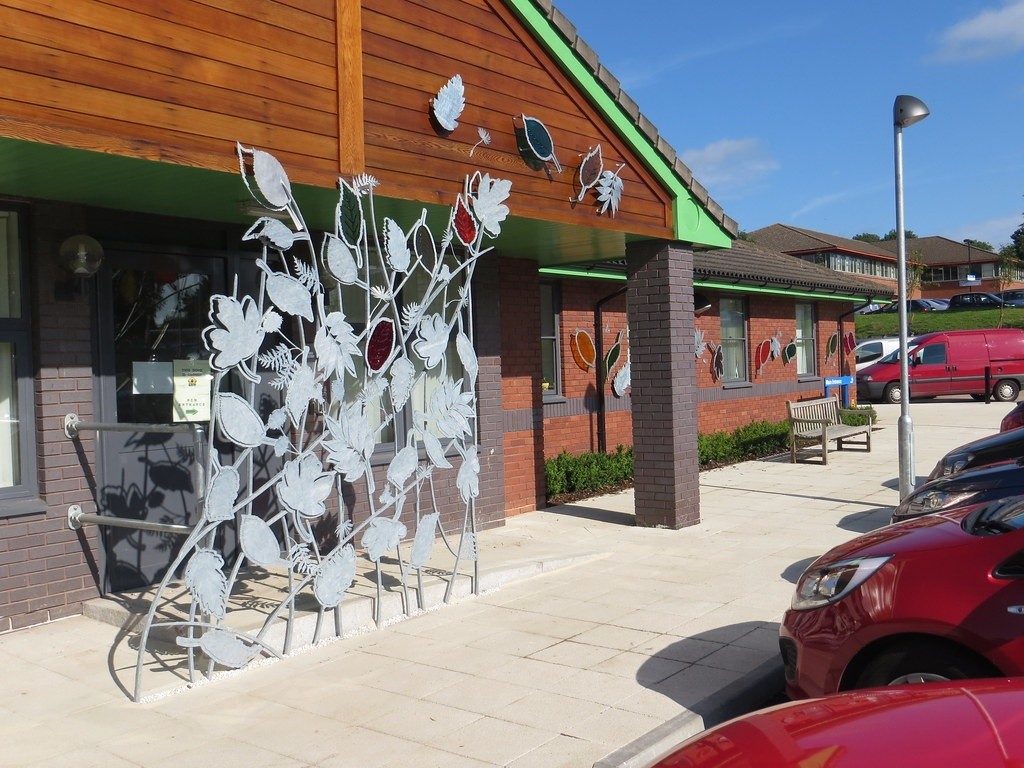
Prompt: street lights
893,93,932,502
964,239,974,292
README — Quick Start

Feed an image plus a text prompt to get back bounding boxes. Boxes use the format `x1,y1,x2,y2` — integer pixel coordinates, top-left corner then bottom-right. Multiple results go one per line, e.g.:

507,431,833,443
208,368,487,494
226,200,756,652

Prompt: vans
856,328,1024,404
852,335,923,374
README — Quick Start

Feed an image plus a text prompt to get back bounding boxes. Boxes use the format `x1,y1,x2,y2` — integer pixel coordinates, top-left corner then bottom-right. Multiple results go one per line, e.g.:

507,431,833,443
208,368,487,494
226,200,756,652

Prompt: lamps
694,291,712,314
53,234,103,301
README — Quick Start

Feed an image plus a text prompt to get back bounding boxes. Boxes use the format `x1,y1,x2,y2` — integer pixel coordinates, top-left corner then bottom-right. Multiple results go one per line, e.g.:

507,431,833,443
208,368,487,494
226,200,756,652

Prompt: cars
779,398,1024,701
863,288,1024,316
652,673,1023,768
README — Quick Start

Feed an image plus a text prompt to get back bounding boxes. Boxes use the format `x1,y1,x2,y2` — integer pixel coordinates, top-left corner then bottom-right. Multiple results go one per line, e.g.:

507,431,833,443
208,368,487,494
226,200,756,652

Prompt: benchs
785,393,875,465
